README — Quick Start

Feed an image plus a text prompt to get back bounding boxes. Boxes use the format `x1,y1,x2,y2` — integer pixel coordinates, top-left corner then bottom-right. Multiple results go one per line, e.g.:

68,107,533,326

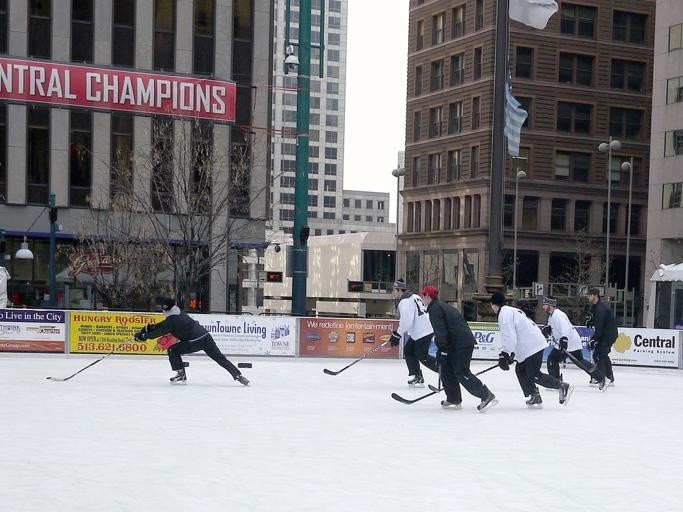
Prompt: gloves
498,352,515,371
436,349,448,365
134,326,147,343
390,331,400,347
585,311,593,328
541,325,552,338
586,337,599,351
558,336,567,353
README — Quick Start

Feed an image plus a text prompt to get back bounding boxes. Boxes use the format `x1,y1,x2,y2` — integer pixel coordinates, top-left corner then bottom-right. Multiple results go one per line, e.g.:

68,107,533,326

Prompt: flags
504,63,529,159
508,0,559,30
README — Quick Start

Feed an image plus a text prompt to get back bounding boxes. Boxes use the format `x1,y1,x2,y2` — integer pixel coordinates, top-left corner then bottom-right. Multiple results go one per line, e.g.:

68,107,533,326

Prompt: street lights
623,156,635,328
600,136,622,304
512,170,527,302
45,191,62,309
390,164,405,281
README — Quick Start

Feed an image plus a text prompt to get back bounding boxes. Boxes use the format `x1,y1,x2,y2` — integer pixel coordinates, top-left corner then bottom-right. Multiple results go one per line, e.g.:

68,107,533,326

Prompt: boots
477,383,496,410
551,379,569,404
170,369,187,383
237,375,249,386
407,370,425,384
524,387,542,405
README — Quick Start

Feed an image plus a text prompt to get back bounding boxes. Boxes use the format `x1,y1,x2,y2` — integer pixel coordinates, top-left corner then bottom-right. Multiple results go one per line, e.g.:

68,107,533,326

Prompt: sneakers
441,398,460,406
589,372,615,390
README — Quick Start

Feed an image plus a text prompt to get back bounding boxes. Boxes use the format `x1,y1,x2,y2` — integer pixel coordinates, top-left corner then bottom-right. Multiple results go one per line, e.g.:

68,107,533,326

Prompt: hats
393,278,408,291
542,295,558,307
417,285,439,298
161,298,175,311
584,288,601,296
489,290,507,306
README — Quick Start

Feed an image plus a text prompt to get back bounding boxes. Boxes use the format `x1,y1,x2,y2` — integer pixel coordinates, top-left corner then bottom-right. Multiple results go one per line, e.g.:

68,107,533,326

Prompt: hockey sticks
546,333,605,391
46,337,136,382
391,365,500,405
324,342,390,375
589,326,593,384
428,362,442,394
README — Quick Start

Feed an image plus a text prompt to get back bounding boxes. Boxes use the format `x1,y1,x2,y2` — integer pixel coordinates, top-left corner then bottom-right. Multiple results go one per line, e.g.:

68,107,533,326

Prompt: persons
584,288,618,386
135,297,250,385
490,291,575,408
421,285,499,413
389,278,440,388
541,296,611,392
